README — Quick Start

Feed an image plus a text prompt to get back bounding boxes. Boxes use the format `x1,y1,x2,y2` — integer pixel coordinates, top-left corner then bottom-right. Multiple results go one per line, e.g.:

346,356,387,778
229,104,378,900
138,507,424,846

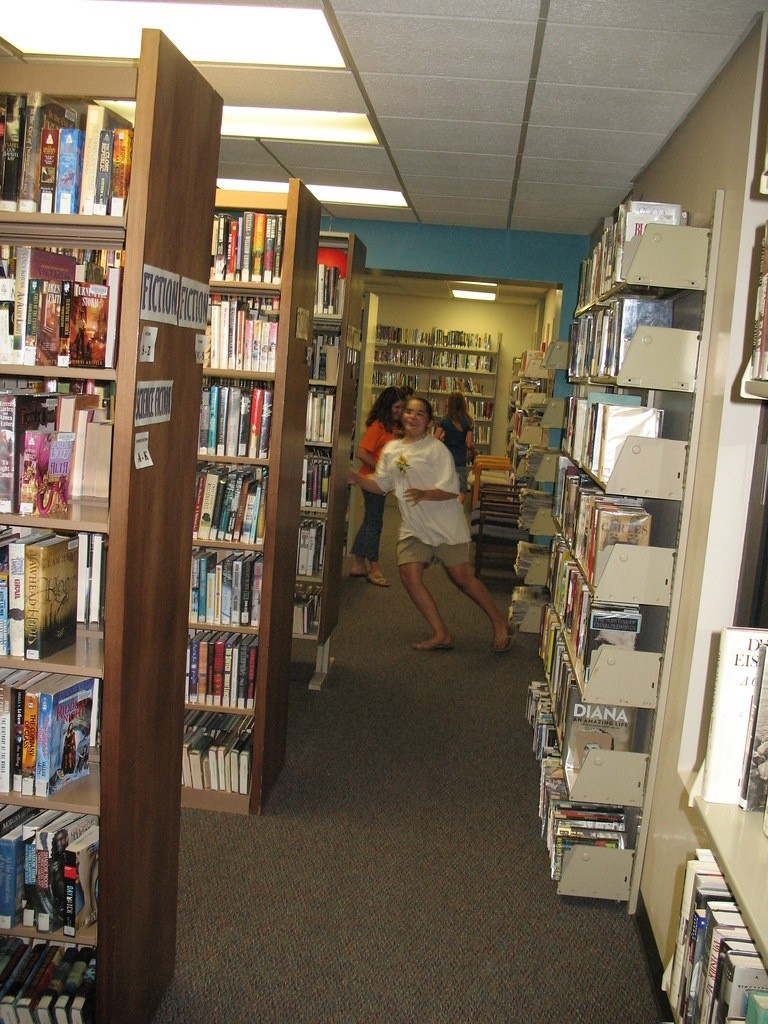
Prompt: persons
433,392,475,504
348,397,513,649
350,386,414,586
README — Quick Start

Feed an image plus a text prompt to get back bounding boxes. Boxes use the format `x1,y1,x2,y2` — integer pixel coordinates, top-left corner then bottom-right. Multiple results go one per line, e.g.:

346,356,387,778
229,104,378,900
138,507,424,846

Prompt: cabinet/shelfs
0,28,224,1023
202,230,368,691
506,357,554,502
667,381,768,1024
0,178,322,818
372,325,503,471
342,293,380,577
515,188,726,917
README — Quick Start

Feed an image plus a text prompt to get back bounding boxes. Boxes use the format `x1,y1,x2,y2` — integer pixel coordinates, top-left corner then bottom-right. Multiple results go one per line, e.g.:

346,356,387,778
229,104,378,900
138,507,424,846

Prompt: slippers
492,623,519,651
412,641,454,650
366,574,390,586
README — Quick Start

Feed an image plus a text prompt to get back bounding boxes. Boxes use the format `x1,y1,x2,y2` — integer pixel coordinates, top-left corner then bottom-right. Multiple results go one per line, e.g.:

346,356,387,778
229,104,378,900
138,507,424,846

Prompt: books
669,628,768,1024
293,264,346,636
508,203,687,880
0,91,133,1024
373,325,493,455
178,211,286,795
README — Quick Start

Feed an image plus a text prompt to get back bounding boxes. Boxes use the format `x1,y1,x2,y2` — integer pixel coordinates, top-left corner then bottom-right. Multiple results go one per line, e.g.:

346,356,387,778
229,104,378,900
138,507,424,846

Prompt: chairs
467,455,542,587
